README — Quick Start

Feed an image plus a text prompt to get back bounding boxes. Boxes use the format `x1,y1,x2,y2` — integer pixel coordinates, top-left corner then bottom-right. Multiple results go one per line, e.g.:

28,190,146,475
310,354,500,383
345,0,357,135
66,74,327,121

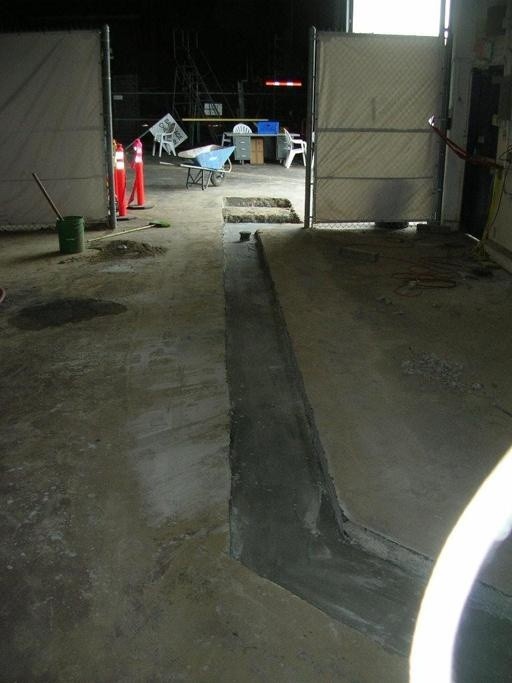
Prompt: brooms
86,221,169,242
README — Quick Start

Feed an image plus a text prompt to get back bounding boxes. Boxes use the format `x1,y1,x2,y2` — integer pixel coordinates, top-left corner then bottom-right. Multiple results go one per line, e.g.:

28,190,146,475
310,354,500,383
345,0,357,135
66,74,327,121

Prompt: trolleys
159,143,236,190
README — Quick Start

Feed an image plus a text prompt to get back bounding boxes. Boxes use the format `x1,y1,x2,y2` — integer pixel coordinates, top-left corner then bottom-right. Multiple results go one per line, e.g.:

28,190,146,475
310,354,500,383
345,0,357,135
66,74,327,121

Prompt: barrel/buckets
57,216,85,253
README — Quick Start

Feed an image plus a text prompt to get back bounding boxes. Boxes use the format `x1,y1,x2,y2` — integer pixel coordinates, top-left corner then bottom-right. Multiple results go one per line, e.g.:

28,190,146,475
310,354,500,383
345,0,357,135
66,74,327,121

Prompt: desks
225,132,300,166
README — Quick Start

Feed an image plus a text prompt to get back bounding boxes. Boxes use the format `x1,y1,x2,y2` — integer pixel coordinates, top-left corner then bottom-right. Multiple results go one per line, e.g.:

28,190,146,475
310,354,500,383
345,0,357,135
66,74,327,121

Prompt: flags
151,112,189,153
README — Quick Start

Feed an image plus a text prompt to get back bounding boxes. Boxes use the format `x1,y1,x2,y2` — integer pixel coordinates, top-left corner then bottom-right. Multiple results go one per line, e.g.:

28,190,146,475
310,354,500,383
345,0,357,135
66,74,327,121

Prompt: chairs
283,127,307,168
152,122,177,157
221,123,252,146
